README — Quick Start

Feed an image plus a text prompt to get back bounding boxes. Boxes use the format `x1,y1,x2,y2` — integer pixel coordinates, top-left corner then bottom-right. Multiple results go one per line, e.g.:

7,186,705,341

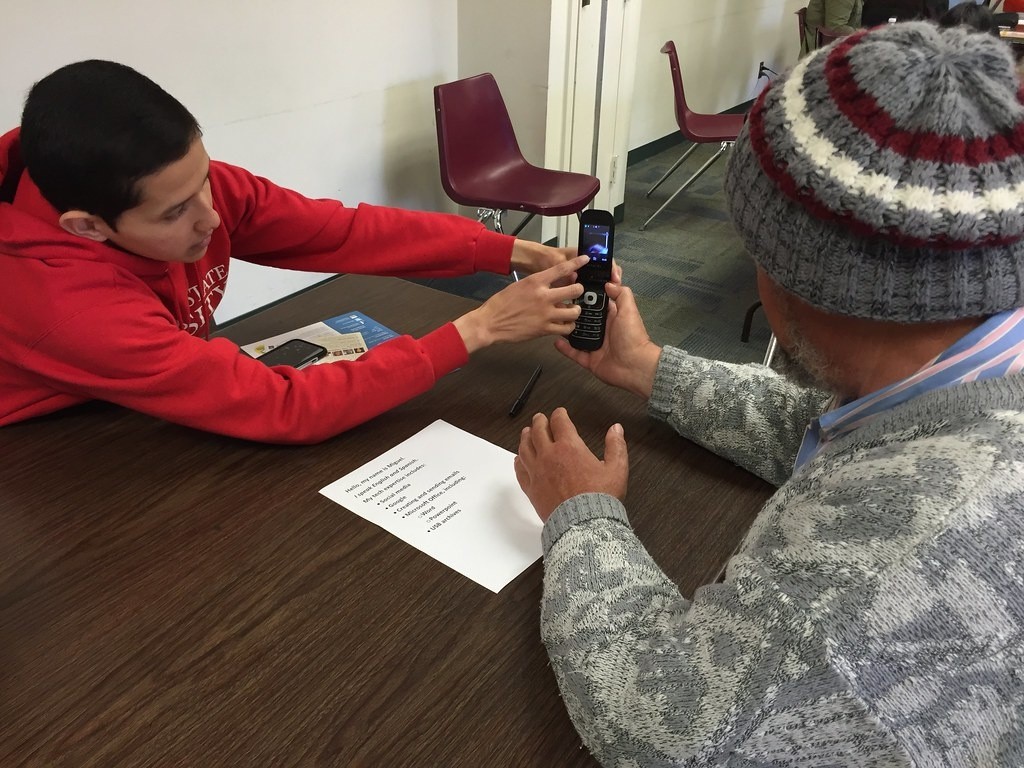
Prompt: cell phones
255,338,327,371
568,209,614,352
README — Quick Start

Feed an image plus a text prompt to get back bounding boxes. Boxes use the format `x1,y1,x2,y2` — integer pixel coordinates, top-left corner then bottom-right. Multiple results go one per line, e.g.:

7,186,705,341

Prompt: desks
0,274,779,768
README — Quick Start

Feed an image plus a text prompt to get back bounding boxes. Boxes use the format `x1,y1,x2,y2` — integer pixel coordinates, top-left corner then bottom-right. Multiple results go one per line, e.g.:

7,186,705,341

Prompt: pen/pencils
508,362,545,415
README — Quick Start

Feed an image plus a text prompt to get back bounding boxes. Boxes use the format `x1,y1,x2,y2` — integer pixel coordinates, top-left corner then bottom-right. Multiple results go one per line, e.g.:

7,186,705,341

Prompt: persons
796,0,1024,63
511,17,1024,768
0,57,625,448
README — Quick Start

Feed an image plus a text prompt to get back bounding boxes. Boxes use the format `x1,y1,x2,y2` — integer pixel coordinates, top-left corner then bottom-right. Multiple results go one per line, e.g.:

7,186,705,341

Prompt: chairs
640,41,745,230
434,73,600,282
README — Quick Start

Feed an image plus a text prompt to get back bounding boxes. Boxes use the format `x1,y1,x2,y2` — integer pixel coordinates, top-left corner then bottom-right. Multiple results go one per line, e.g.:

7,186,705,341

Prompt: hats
724,18,1024,324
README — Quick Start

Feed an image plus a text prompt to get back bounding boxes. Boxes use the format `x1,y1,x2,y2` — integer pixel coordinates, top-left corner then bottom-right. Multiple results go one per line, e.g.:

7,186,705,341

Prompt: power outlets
758,62,764,79
